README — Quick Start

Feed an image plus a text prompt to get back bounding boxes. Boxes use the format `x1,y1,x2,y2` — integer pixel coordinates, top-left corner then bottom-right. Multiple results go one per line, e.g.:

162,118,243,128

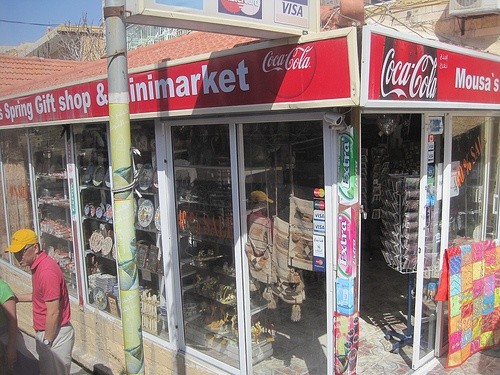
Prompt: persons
0,280,19,375
247,190,272,239
5,229,75,375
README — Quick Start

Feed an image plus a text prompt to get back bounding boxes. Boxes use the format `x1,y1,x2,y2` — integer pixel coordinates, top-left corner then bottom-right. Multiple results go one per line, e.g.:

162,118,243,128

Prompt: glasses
15,244,35,255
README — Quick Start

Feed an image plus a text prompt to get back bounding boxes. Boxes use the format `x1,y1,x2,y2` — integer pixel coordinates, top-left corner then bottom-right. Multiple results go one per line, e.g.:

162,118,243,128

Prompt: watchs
43,339,52,345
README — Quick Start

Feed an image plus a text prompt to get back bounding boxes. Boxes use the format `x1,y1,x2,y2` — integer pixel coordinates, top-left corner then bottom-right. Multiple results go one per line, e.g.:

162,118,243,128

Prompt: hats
249,190,274,203
4,228,40,253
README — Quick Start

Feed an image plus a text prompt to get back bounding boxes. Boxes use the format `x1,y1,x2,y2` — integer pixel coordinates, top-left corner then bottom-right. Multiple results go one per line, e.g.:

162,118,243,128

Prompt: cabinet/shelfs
378,174,440,354
175,165,286,367
